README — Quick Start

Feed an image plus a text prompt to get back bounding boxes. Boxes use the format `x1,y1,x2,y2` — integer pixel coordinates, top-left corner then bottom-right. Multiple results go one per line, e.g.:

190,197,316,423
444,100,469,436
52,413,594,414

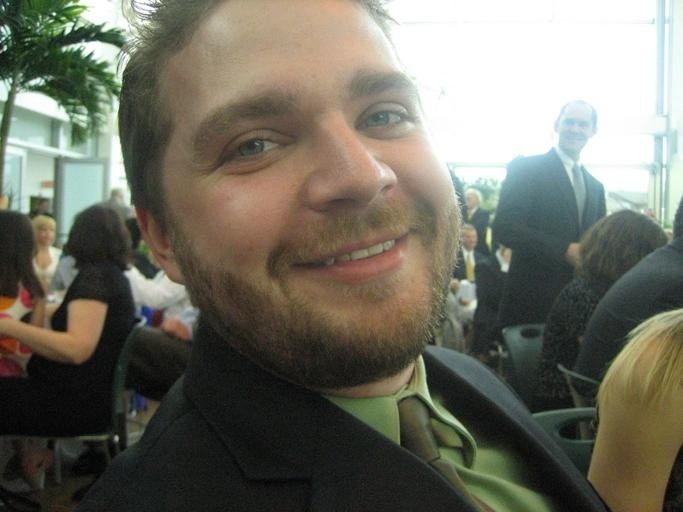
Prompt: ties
466,253,474,280
398,395,496,511
572,163,584,226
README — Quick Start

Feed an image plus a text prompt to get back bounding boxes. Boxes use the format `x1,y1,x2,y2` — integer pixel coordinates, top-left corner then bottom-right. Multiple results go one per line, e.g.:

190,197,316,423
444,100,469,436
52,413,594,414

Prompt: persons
450,169,513,360
73,0,611,512
1,188,201,511
495,102,606,342
535,196,683,511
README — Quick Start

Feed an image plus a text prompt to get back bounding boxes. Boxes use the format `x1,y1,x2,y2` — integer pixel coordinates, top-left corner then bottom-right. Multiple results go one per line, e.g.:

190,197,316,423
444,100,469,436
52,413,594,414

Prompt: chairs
0,314,147,491
555,361,601,442
530,406,599,479
501,323,544,399
